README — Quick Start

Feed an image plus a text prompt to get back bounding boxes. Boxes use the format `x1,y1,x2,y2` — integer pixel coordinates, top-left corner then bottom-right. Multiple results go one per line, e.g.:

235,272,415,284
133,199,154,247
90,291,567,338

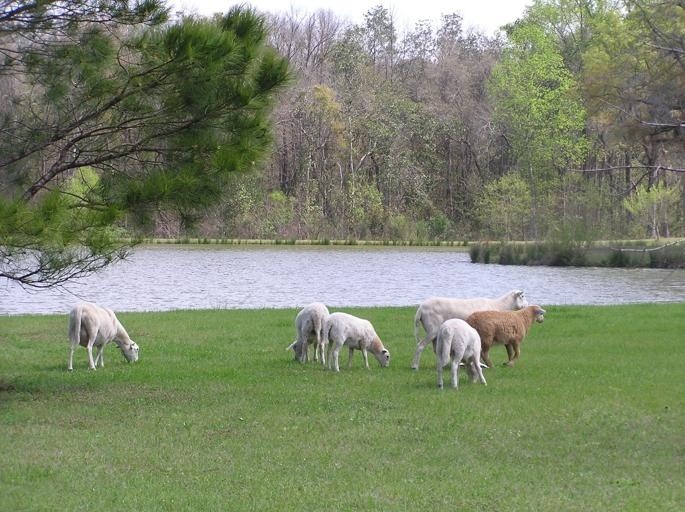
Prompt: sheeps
283,303,330,366
66,300,142,373
408,288,546,392
322,310,391,375
